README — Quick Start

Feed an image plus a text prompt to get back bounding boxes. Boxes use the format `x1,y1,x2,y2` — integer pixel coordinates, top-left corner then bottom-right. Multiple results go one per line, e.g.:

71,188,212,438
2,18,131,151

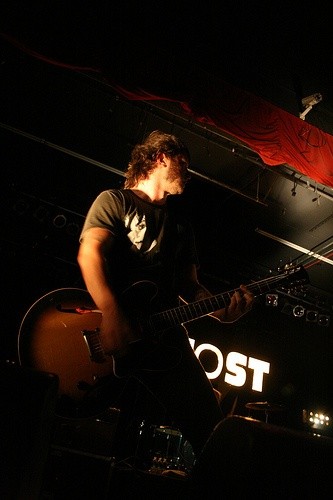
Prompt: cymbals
244,401,289,412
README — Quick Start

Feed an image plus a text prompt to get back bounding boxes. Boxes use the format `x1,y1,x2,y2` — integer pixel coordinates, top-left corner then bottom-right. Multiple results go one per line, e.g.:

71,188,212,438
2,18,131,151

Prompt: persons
76,130,254,500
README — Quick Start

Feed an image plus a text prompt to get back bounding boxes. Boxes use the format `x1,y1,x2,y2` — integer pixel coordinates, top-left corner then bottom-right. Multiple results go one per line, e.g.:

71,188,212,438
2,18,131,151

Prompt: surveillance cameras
301,93,323,106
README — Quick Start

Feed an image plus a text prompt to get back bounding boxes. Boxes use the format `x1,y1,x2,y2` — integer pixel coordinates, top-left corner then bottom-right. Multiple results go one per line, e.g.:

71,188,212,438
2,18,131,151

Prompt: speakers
186,416,333,500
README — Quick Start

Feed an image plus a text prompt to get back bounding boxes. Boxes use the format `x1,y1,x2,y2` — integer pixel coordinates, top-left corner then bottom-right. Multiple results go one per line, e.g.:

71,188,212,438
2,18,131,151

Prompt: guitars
18,257,311,419
